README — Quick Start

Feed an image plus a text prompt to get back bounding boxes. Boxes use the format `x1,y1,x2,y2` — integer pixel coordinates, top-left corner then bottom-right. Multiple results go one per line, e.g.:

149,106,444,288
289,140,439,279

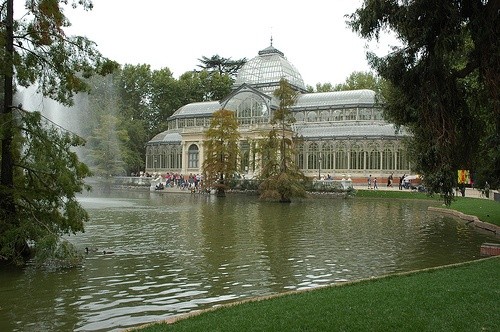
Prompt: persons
132,171,204,191
484,181,490,199
372,178,378,190
367,175,373,189
387,173,425,192
460,180,466,197
324,174,333,180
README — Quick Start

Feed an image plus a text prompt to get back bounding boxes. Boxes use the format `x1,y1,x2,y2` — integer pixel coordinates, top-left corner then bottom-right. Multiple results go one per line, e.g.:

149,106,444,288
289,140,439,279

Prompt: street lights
155,158,157,172
319,157,323,180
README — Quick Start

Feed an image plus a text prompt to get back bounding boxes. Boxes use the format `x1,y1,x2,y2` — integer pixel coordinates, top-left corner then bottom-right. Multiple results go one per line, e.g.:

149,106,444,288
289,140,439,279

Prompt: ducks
83,246,114,258
